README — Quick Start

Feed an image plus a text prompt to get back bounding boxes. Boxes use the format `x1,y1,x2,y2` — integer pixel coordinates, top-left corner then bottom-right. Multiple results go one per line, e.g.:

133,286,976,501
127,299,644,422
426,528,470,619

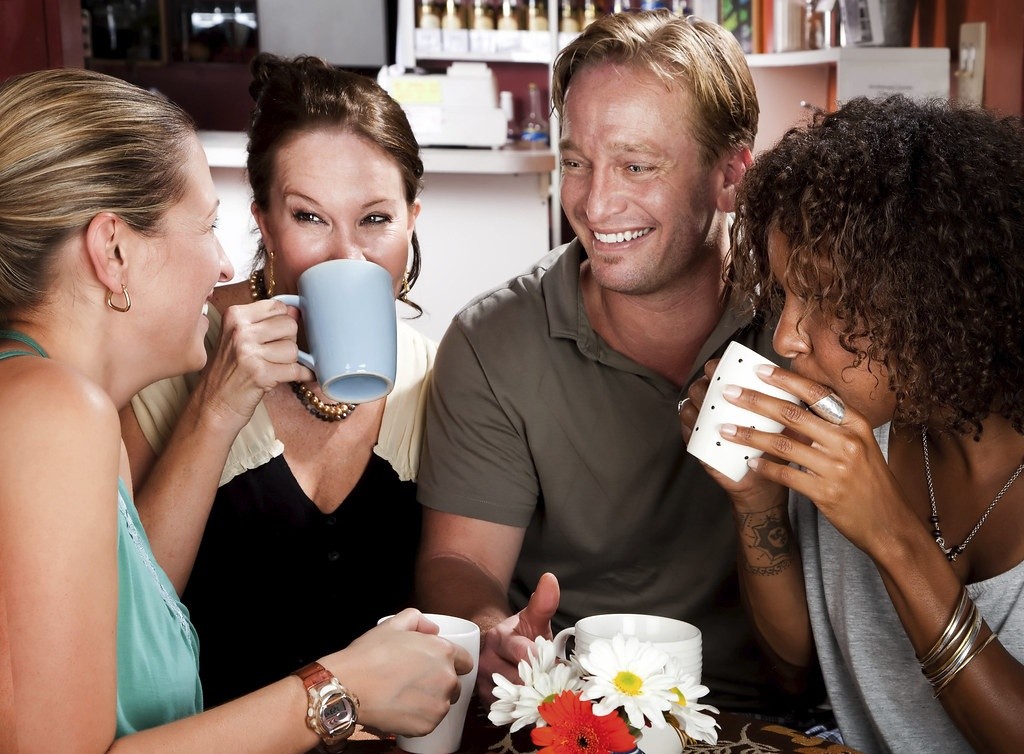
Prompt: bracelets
916,583,999,694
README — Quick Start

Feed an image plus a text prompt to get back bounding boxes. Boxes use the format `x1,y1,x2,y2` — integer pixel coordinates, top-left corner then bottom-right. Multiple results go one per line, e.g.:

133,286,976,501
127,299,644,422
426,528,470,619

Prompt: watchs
293,661,361,747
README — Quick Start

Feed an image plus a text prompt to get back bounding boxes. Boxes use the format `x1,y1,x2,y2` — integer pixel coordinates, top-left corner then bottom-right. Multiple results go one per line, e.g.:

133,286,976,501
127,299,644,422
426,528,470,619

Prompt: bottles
498,82,551,151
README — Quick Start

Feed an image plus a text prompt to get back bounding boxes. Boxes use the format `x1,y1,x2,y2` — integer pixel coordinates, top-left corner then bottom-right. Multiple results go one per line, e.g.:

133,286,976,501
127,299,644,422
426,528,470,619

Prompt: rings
807,391,845,426
677,397,690,413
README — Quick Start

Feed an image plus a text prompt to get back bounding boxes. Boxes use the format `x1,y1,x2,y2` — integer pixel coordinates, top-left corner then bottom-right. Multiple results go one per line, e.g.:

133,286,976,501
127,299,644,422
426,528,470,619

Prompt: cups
686,340,801,482
376,613,479,754
552,614,703,754
271,259,397,404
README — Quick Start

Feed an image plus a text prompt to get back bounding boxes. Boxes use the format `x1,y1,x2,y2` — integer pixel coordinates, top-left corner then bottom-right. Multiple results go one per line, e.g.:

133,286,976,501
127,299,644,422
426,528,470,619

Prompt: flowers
487,633,722,754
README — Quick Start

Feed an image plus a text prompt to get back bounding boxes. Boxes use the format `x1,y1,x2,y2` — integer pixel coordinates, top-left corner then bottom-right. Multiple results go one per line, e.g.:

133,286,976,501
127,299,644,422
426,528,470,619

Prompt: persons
677,93,1024,754
419,10,791,717
0,65,474,754
116,52,445,754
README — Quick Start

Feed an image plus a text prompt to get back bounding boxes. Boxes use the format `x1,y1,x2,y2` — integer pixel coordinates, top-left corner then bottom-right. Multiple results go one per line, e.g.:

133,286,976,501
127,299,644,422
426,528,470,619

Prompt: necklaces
248,269,362,421
923,419,1024,562
